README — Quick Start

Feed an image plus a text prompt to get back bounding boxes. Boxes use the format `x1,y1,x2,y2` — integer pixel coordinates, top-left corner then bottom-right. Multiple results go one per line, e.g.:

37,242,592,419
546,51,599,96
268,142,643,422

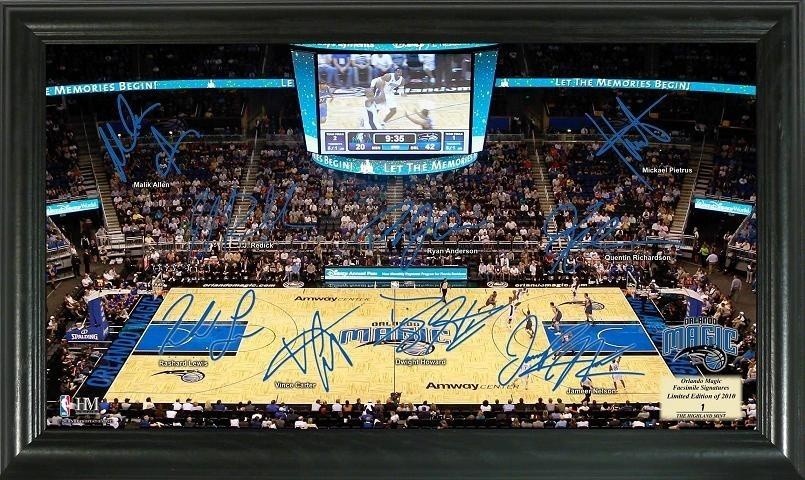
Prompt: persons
627,289,758,430
584,292,597,325
43,287,137,428
569,272,579,297
438,277,449,303
579,371,596,406
508,288,535,338
608,355,626,391
476,291,497,313
548,301,563,336
552,330,576,360
